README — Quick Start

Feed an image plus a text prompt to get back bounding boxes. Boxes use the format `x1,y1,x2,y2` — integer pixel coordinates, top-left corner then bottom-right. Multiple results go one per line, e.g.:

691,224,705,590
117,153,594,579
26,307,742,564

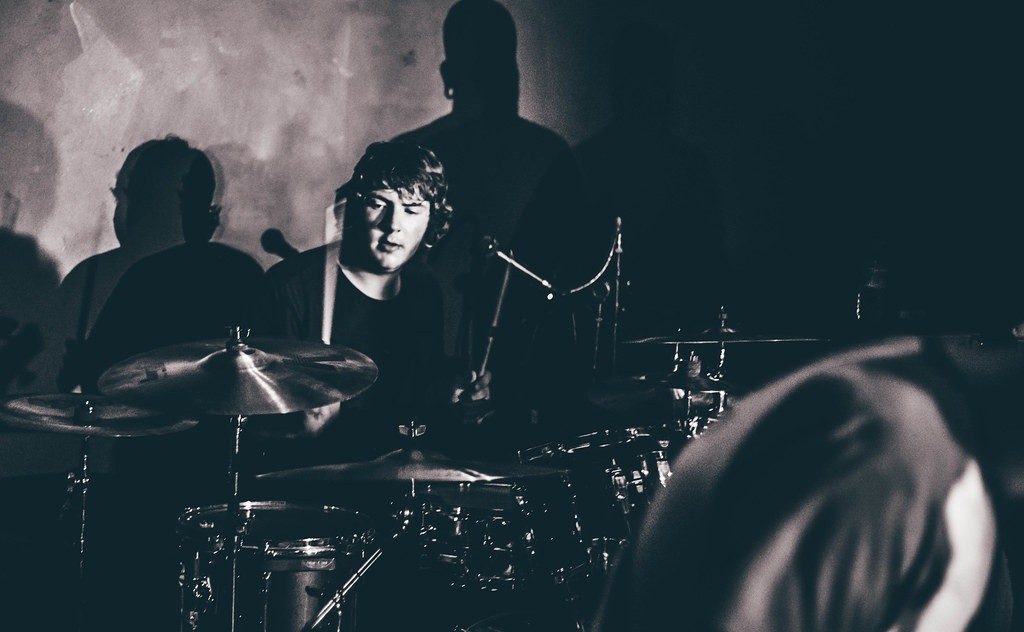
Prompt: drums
383,504,553,632
522,414,699,571
584,373,735,433
176,500,371,632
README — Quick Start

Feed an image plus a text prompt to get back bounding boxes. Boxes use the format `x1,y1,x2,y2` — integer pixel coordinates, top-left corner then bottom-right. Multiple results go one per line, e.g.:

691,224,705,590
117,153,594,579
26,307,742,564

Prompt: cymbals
98,334,379,416
0,393,198,439
623,329,823,345
255,444,571,488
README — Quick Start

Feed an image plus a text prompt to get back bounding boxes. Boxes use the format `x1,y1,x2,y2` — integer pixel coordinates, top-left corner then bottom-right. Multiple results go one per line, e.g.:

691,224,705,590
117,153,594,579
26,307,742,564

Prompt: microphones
260,228,300,258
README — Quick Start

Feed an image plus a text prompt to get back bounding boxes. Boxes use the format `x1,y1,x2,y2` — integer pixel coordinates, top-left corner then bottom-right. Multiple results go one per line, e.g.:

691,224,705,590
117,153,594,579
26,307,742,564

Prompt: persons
488,19,736,445
223,134,500,514
591,305,1024,632
